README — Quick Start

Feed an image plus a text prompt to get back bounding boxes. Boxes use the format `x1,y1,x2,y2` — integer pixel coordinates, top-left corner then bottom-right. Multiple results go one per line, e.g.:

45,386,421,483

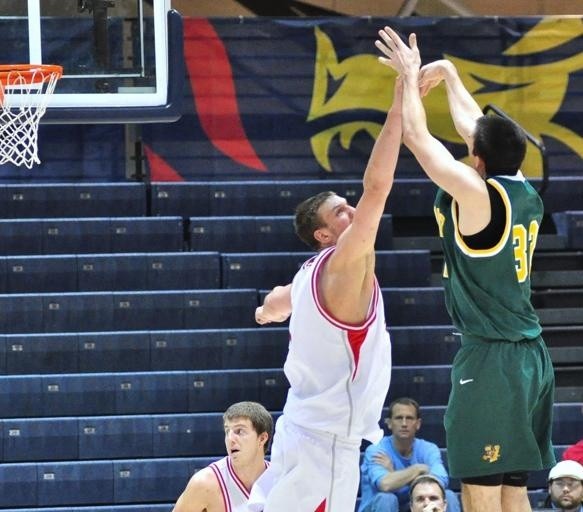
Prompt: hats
547,460,583,482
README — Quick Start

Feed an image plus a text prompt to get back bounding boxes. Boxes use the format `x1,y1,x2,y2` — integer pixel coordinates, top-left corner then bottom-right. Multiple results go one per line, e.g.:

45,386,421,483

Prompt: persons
241,70,405,512
168,399,271,512
374,22,555,512
356,396,461,512
536,460,582,512
407,474,448,512
